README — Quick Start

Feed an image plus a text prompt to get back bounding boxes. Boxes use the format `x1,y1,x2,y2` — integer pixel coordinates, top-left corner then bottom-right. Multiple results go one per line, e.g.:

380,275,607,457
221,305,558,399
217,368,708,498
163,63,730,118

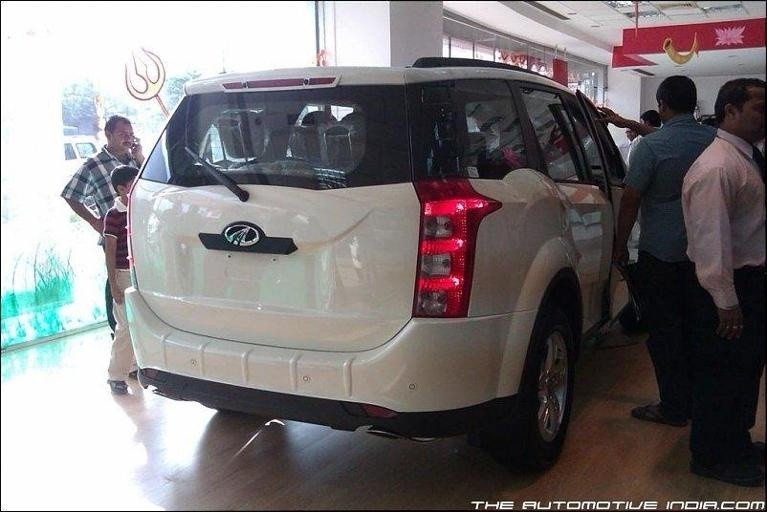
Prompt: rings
740,325,746,328
725,325,730,328
732,325,738,329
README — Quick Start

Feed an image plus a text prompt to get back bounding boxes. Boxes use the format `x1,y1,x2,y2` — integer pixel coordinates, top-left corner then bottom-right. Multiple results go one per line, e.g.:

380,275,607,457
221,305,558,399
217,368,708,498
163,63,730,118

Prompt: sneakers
110,381,128,394
688,441,767,487
631,404,688,427
129,370,138,380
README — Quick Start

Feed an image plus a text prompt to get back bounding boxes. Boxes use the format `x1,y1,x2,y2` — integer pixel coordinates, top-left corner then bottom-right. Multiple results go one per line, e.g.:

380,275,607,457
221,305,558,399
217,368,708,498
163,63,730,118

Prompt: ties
752,144,767,184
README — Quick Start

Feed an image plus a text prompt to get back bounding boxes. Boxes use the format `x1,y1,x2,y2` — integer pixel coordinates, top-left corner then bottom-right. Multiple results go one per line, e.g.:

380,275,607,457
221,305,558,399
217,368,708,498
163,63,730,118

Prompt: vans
61,137,104,173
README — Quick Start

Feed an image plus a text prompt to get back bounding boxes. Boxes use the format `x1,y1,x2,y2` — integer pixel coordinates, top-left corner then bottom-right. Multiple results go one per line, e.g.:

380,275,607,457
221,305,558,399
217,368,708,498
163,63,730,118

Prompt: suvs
122,57,631,469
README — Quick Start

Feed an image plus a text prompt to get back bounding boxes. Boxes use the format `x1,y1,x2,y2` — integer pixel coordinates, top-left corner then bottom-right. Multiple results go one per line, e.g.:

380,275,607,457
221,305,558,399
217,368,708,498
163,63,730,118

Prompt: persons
626,110,662,249
103,165,140,393
681,77,766,487
625,129,638,167
574,121,603,175
62,116,147,338
593,76,713,426
